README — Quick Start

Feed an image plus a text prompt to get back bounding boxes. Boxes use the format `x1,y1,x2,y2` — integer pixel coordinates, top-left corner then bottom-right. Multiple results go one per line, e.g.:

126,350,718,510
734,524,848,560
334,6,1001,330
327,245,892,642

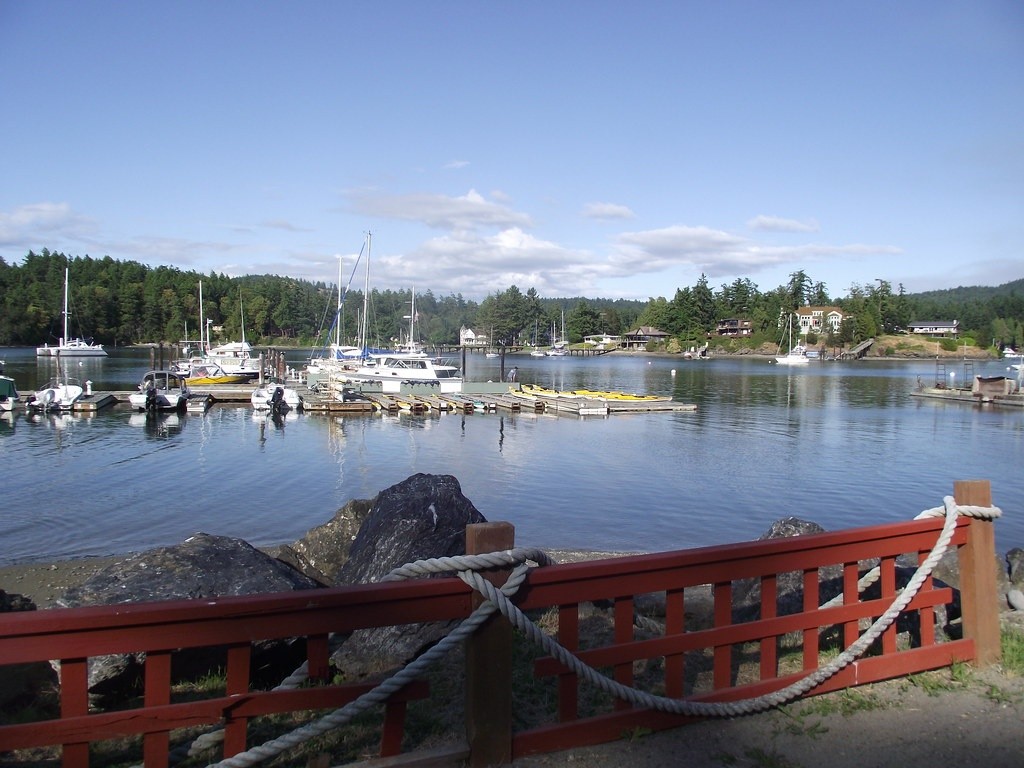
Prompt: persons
506,367,518,382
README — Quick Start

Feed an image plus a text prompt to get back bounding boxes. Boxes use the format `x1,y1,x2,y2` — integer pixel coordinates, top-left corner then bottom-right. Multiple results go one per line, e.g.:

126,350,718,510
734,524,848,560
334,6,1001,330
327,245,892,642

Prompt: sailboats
774,313,809,363
209,287,254,353
546,310,569,356
531,319,546,357
306,229,463,394
166,280,270,378
485,325,499,358
1011,343,1024,370
35,268,108,357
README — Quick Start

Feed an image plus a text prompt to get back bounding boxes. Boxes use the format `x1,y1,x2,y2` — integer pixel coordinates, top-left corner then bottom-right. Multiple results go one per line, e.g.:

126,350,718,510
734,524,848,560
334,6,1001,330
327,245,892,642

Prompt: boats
698,345,710,360
0,374,19,412
167,363,254,386
684,352,691,359
361,393,487,411
250,382,302,412
129,370,190,412
24,375,83,413
509,382,673,402
